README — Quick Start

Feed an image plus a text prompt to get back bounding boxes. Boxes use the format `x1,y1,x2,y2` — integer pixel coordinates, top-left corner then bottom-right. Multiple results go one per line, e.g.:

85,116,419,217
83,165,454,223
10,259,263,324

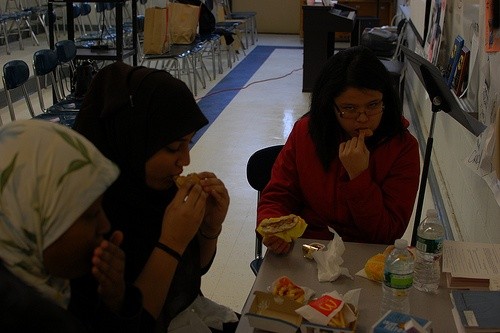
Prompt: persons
70,64,242,333
0,119,126,333
256,46,420,256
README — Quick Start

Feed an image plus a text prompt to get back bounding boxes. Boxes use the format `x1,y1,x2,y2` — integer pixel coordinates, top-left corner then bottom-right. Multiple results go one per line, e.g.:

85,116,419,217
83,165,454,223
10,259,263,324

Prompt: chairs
0,0,259,131
247,144,285,276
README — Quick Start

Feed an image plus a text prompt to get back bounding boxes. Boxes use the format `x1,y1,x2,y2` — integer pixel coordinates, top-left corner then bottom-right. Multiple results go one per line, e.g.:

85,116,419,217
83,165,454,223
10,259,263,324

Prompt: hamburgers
173,173,204,196
261,214,298,234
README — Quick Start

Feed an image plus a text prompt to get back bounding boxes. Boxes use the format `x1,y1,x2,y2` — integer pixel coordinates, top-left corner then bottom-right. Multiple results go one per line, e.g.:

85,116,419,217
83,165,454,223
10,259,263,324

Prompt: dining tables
234,237,499,333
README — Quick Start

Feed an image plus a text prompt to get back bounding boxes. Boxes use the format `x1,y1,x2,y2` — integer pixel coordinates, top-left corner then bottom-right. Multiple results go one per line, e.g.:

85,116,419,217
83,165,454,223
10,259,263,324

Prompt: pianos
302,1,359,94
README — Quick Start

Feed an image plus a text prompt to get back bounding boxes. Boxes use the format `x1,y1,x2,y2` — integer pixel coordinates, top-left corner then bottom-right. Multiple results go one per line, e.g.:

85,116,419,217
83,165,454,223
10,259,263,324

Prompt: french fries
327,310,348,329
271,282,301,300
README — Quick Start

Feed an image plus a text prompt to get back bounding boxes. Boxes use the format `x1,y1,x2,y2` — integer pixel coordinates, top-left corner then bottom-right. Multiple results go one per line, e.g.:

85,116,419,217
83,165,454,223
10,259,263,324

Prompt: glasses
332,98,386,120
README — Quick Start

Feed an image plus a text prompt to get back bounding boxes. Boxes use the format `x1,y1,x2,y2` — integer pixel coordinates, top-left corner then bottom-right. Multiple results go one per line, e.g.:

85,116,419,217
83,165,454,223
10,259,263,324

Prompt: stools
378,60,408,115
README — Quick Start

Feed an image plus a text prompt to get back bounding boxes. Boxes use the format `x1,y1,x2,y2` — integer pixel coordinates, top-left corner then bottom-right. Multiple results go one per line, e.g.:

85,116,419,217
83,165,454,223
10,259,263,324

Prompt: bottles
412,209,444,292
380,239,414,318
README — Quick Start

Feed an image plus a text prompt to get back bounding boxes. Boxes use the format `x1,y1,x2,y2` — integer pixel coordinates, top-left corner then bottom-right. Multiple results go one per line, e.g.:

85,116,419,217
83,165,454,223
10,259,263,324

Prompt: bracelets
197,227,222,239
153,241,181,260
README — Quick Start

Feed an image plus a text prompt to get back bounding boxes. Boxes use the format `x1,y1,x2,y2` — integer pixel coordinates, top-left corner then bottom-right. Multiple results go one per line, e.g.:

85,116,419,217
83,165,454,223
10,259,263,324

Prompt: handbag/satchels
143,1,174,55
167,0,203,44
73,59,99,98
